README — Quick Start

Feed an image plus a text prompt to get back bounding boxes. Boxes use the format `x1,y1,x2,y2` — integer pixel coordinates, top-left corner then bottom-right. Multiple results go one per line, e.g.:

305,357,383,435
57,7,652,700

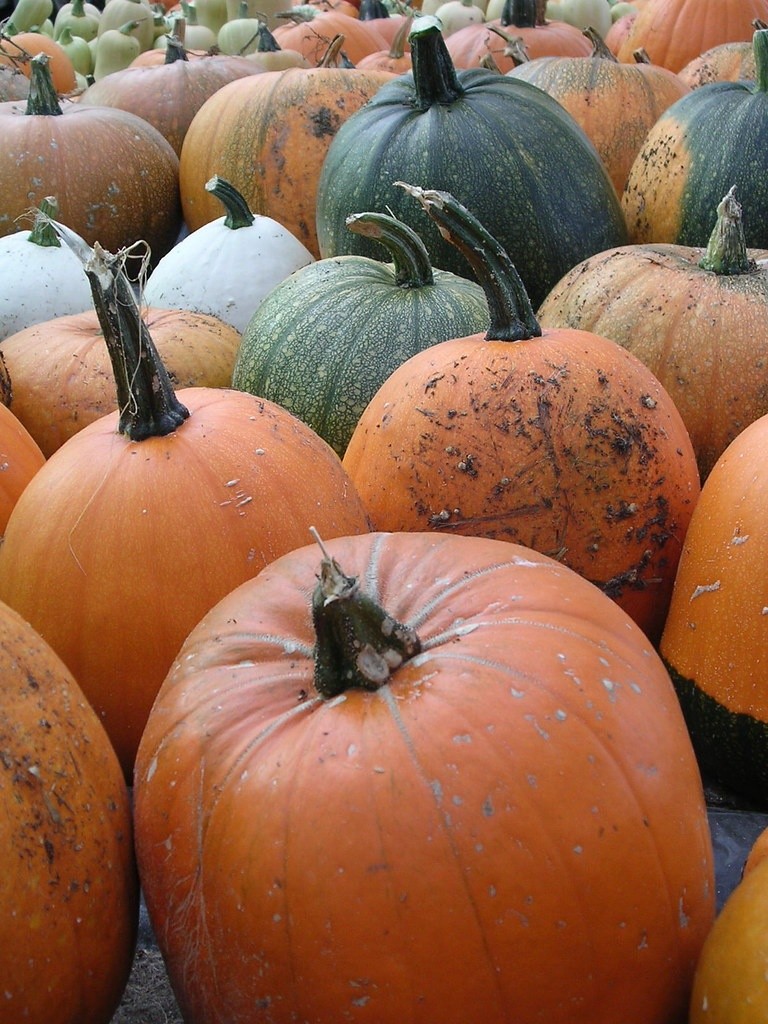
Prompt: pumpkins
0,0,768,1024
